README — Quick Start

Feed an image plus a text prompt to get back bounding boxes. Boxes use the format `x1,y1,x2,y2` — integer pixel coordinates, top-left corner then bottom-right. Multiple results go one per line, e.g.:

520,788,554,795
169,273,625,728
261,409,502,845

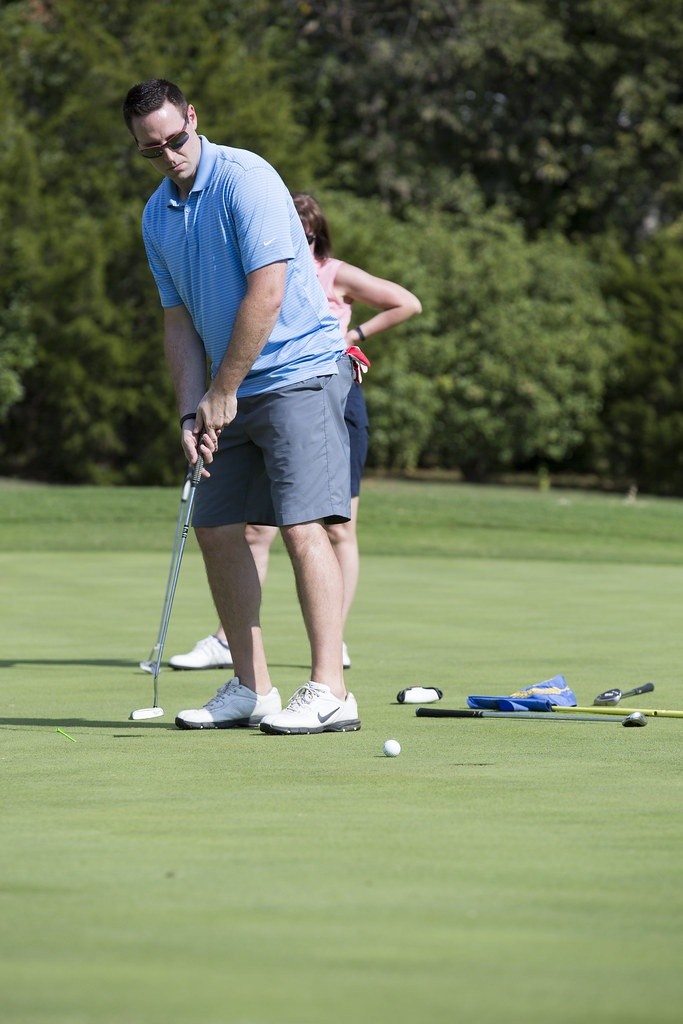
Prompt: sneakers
168,635,234,669
260,681,361,735
175,677,282,729
342,641,350,669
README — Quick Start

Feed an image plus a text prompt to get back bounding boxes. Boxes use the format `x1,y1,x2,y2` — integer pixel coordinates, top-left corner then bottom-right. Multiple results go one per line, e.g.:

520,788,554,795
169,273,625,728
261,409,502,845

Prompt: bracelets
355,325,366,341
180,413,196,429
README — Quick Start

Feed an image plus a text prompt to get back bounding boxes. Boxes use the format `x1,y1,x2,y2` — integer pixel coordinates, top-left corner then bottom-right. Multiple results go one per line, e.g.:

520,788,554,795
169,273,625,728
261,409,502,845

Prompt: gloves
345,345,371,384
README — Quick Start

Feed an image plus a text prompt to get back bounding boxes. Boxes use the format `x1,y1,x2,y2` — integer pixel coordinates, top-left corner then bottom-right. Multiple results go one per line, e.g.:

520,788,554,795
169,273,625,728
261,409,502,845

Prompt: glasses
134,111,189,158
306,234,316,245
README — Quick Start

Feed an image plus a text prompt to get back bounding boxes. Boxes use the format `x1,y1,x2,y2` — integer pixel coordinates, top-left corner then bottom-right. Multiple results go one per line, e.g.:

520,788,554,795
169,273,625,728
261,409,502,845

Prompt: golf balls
382,739,401,757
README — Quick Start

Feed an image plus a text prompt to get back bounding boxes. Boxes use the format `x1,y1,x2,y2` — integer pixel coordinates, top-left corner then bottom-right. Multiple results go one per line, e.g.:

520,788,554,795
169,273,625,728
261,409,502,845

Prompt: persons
123,80,360,732
170,193,422,668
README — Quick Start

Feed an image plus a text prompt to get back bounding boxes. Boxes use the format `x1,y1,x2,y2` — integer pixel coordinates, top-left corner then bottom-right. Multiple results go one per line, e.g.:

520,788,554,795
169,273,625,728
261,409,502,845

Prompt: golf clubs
415,706,648,728
138,463,195,674
593,682,655,706
128,422,209,720
